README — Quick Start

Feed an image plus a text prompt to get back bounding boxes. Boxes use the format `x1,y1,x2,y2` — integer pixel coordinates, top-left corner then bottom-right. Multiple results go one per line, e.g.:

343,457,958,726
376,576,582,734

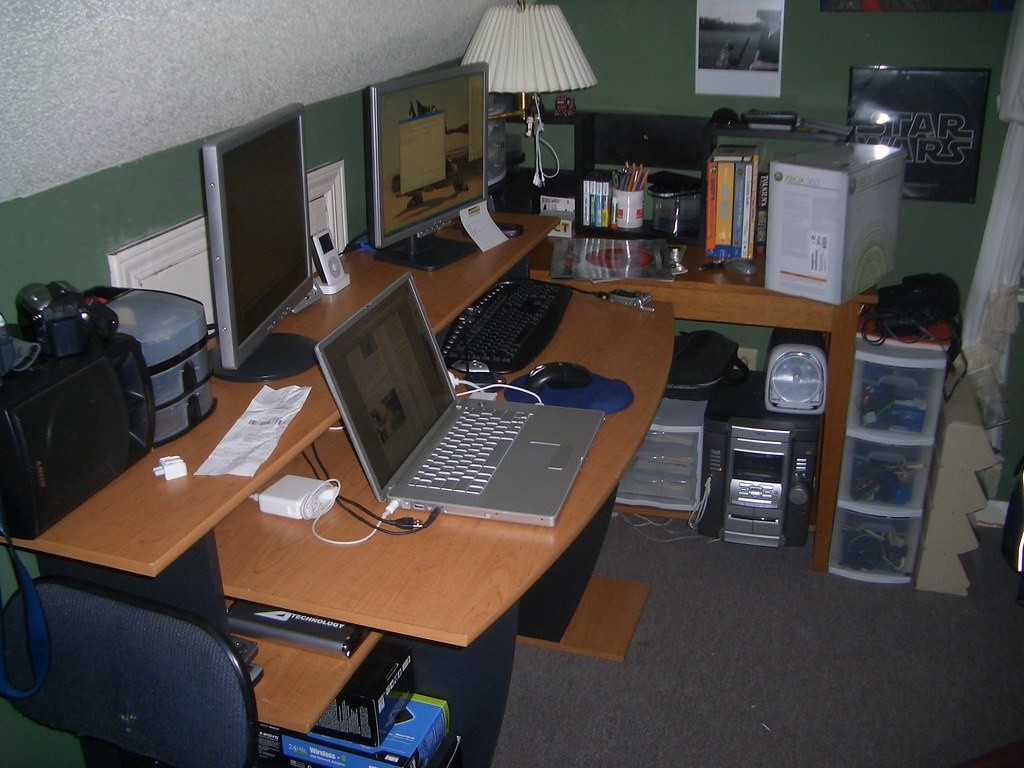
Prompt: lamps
459,0,597,120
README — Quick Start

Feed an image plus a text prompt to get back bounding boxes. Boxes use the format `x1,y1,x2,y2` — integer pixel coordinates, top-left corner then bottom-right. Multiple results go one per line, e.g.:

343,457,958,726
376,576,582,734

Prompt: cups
612,187,644,228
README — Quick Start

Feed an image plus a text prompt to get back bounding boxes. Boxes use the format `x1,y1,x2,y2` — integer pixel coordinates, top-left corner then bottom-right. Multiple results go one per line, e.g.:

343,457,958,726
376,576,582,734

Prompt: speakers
0,331,155,541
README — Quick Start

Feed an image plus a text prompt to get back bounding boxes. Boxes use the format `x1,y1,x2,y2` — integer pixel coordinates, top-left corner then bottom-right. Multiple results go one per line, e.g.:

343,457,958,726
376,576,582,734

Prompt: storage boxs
79,287,217,447
255,696,449,768
311,639,415,748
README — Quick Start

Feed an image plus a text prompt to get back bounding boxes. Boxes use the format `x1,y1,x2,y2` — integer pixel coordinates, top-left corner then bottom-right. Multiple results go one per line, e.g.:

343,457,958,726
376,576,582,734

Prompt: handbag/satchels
663,329,749,402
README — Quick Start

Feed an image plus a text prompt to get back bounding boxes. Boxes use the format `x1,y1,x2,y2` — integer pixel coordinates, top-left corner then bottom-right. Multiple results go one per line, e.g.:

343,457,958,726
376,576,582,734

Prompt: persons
716,12,780,71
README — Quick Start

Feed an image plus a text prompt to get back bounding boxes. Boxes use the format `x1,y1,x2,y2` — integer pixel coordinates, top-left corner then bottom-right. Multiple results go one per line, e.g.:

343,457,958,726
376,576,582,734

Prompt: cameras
16,281,91,359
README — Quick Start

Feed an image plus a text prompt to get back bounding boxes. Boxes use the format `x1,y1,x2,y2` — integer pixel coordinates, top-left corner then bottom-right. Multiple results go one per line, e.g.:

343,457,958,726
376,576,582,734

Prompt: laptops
314,270,607,526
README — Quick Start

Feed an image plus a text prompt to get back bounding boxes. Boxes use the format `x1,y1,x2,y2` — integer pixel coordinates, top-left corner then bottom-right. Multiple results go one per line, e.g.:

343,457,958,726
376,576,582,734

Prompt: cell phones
609,288,656,312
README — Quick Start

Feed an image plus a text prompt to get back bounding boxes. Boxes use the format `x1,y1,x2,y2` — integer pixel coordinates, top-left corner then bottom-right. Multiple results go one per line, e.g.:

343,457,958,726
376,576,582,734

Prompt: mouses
526,362,592,390
723,259,758,276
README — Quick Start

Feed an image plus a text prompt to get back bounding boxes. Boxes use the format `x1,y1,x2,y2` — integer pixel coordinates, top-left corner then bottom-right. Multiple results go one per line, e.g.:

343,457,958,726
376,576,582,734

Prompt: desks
528,233,881,574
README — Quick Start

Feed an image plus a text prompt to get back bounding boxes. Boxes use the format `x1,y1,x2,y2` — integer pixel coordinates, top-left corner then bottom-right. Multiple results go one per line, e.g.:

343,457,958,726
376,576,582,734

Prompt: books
583,169,612,228
548,238,688,283
706,142,768,259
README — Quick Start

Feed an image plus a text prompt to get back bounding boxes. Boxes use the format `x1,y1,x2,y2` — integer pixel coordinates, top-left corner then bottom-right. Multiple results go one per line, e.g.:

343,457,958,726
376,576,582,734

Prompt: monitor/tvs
201,102,320,382
362,61,490,271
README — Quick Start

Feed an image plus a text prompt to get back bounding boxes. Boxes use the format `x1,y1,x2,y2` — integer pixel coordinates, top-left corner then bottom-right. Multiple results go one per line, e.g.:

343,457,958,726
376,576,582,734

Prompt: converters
258,474,333,520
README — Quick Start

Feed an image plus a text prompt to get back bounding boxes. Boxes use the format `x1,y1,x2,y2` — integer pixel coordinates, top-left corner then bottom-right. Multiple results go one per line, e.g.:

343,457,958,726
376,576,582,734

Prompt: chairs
0,580,257,768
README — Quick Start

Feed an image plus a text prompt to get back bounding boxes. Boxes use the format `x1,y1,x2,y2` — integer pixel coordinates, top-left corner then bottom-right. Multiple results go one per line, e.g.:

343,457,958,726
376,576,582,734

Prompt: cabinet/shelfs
0,214,676,736
828,316,951,584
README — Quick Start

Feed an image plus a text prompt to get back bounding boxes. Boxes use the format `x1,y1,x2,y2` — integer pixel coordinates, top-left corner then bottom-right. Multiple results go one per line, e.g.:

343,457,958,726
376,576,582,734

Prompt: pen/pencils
613,160,651,191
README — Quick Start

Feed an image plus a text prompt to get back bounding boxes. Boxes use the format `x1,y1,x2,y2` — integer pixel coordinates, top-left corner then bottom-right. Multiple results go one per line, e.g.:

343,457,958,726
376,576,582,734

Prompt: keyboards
437,277,573,373
